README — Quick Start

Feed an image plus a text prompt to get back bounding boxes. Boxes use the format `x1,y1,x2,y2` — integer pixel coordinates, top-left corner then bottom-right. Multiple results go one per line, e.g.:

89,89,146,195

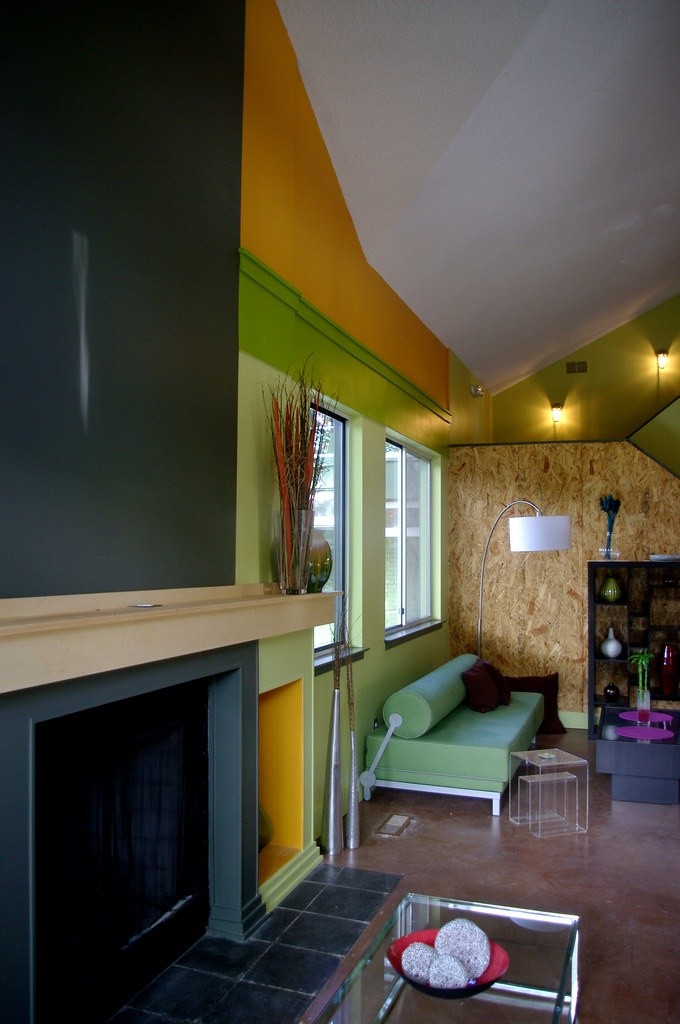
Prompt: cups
663,644,672,659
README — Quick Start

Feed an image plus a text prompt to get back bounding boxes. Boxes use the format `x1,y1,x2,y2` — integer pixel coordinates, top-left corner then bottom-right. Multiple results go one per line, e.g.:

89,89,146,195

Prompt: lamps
655,350,668,369
509,511,571,553
550,403,563,422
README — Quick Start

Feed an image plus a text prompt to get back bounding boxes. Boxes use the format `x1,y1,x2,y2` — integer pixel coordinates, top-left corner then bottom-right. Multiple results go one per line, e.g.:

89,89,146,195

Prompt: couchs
364,691,544,815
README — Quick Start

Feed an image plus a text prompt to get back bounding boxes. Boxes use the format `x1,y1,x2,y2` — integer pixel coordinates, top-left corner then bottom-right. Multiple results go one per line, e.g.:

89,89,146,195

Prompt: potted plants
626,647,655,723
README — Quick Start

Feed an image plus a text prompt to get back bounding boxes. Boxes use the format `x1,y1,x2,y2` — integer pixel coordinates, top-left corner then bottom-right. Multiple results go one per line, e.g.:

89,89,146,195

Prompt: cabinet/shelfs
587,560,680,742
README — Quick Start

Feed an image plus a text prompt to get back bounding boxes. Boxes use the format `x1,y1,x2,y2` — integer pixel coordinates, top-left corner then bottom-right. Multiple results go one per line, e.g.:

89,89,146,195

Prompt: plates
648,554,680,560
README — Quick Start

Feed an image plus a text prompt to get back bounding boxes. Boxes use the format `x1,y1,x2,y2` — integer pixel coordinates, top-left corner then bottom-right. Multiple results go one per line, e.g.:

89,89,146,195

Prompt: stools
509,747,589,839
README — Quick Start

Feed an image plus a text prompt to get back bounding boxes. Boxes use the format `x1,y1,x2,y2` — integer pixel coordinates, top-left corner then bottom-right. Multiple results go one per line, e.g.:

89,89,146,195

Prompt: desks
596,705,680,805
312,892,581,1024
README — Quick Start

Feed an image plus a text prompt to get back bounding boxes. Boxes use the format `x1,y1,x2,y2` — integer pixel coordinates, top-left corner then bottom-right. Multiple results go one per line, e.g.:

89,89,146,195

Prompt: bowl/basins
386,930,511,999
